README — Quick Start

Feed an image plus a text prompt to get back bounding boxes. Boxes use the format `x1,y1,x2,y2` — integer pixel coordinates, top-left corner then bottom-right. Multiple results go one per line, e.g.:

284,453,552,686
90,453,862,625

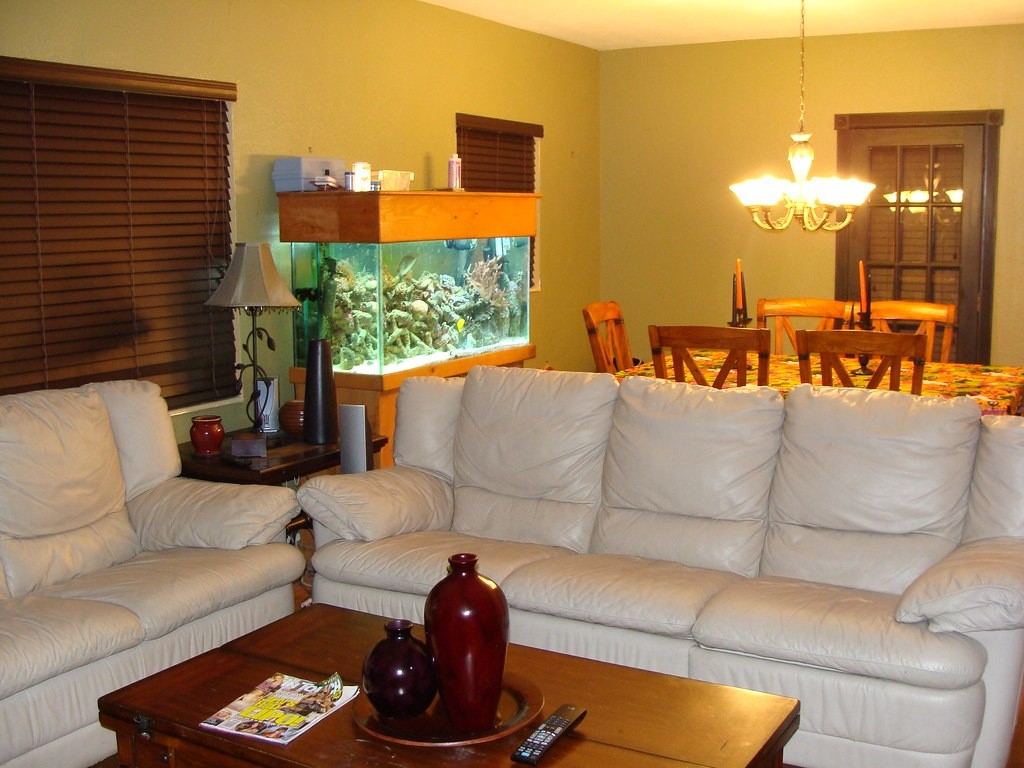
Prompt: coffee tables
98,602,800,768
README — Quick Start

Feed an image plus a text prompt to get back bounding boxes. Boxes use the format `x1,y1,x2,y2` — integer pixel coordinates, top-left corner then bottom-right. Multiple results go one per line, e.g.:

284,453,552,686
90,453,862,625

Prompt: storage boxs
231,432,267,458
275,191,543,472
271,157,346,192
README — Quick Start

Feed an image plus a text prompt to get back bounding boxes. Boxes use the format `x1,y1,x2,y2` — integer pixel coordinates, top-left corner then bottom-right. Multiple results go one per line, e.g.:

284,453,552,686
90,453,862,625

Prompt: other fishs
456,319,466,333
397,253,418,277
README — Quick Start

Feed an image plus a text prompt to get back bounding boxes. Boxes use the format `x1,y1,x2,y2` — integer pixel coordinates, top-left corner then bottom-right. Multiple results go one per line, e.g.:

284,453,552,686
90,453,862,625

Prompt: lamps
201,242,302,451
885,147,963,228
728,1,877,231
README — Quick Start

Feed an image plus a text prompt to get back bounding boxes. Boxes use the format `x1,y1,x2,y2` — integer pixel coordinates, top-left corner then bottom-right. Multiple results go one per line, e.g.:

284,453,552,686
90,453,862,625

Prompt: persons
262,728,288,738
289,691,327,717
260,674,284,697
240,722,259,734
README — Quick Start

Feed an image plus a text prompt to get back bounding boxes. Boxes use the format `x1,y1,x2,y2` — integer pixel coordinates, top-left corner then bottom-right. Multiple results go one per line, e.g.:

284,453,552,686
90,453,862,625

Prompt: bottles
448,154,461,188
361,620,437,719
303,340,337,444
424,553,509,732
352,162,371,192
279,400,304,435
345,172,354,191
190,415,224,456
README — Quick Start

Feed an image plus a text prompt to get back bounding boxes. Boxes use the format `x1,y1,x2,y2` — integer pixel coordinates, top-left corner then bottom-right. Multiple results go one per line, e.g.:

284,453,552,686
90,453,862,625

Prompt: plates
350,672,545,747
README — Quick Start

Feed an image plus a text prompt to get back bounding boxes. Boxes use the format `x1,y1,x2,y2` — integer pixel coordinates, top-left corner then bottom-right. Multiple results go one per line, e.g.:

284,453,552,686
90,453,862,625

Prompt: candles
736,259,743,309
859,261,867,312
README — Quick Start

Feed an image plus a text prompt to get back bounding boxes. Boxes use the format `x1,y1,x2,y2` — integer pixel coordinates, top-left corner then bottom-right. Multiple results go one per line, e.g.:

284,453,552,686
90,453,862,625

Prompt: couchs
0,380,306,768
295,362,1024,768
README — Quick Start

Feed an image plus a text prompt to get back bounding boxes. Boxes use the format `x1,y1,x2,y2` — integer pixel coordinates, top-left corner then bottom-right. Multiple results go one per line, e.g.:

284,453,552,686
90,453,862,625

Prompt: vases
361,618,437,718
190,415,224,457
304,338,339,444
424,552,509,735
278,401,305,435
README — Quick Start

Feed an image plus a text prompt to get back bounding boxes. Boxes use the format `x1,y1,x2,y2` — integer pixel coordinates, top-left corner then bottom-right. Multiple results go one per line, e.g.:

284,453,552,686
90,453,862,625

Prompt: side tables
177,423,389,547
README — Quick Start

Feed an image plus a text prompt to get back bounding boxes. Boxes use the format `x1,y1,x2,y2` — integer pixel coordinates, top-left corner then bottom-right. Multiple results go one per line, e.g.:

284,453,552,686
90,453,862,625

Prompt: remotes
511,704,588,766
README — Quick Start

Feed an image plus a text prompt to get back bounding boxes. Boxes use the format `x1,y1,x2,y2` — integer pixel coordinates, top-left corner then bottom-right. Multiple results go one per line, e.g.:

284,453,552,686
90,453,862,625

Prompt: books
199,672,361,745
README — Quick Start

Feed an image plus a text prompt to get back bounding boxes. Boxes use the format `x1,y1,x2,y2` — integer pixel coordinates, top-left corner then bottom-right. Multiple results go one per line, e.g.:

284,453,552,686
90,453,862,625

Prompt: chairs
854,301,957,363
795,330,928,395
647,325,771,388
757,296,853,357
582,301,634,373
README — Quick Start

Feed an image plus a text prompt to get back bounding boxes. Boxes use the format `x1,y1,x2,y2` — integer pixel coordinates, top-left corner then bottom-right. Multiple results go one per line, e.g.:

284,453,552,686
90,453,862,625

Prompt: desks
614,349,1024,434
